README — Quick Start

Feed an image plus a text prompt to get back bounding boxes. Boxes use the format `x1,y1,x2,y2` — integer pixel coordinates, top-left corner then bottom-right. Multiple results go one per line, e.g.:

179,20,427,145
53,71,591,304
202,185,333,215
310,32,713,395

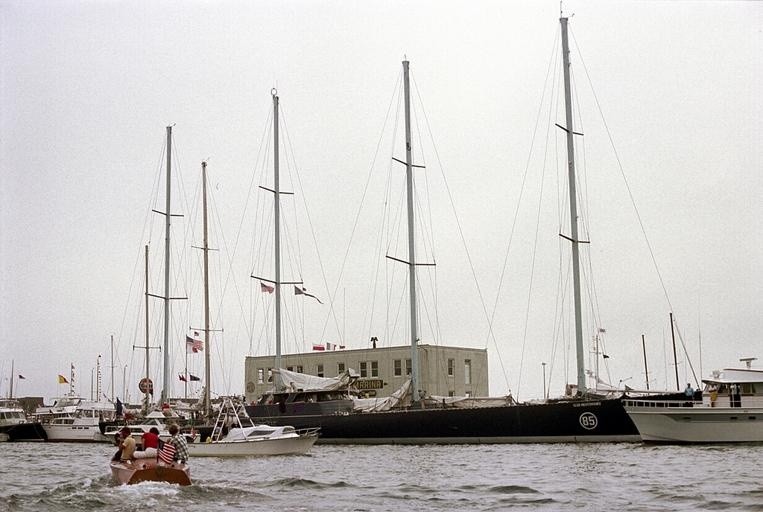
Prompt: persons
132,426,160,458
110,427,136,461
708,381,718,406
161,402,173,417
165,424,189,462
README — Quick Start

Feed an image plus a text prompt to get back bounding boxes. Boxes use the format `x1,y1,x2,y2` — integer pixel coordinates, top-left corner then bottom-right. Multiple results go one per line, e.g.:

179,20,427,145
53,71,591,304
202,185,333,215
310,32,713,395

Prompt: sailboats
177,1,700,445
0,127,208,446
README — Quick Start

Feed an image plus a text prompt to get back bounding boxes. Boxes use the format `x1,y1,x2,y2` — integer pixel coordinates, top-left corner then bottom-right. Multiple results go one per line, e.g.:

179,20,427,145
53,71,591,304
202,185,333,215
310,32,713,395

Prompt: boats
619,358,763,444
108,453,192,490
181,399,322,457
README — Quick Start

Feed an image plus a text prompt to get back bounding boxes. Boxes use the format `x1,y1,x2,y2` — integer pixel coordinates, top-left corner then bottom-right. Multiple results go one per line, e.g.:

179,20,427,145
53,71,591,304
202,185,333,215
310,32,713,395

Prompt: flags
603,354,609,358
260,281,273,294
339,345,344,349
325,342,336,350
191,425,197,440
599,328,605,332
17,374,26,379
684,382,693,404
186,335,202,354
157,437,174,464
58,373,68,384
312,343,326,351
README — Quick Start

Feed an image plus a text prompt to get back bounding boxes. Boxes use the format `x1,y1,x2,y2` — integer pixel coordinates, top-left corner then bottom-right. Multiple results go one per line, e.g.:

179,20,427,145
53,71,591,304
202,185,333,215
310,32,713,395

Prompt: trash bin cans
728,394,741,407
199,427,216,442
